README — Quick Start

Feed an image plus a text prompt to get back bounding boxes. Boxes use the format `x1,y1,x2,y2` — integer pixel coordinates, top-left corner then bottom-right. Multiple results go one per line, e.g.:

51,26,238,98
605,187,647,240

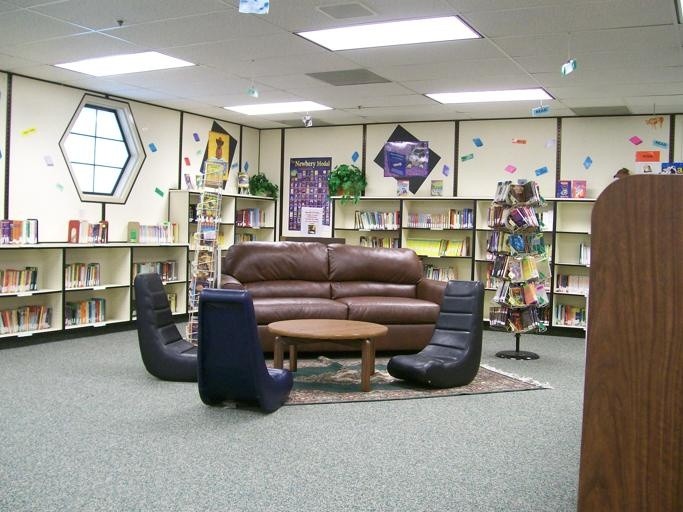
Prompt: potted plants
325,162,367,207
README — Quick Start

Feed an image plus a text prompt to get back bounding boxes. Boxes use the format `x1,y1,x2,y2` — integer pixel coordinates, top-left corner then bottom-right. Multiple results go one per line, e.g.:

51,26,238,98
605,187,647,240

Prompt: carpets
264,357,553,405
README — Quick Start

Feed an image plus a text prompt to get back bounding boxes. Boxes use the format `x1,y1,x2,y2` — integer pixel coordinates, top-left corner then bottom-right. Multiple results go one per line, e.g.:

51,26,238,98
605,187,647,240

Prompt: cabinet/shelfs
169,190,277,323
328,196,595,337
0,239,189,349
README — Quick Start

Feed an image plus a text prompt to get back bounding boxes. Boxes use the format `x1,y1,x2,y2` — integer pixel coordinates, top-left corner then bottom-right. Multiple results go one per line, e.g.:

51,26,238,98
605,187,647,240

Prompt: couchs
220,241,448,352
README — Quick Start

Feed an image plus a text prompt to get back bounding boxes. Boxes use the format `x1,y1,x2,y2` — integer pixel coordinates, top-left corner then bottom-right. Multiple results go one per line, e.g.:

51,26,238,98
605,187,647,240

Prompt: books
486,179,553,333
431,180,443,196
190,203,198,220
235,207,266,244
71,221,108,243
3,305,53,332
167,293,177,312
65,262,100,288
132,260,178,284
359,236,399,248
128,222,174,244
405,237,470,257
190,229,224,248
2,218,37,246
353,210,400,230
397,180,410,196
65,297,106,326
406,208,474,229
556,180,586,199
555,273,589,327
2,267,37,293
579,243,590,265
423,263,458,283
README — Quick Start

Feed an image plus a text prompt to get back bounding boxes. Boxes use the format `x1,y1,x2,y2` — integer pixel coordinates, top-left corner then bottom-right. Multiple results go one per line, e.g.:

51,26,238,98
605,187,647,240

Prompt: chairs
133,273,292,412
386,280,484,389
